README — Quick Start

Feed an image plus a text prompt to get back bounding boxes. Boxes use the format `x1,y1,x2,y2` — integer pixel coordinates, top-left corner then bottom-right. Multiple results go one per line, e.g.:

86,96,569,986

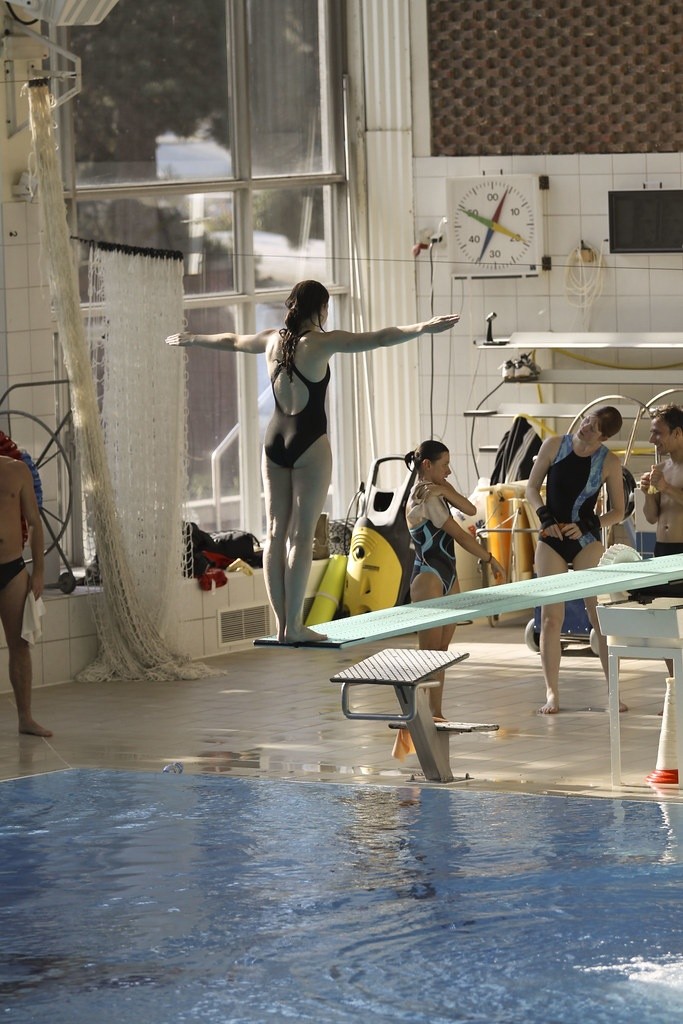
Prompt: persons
165,280,460,643
525,406,628,714
405,440,506,721
640,405,683,716
0,455,54,738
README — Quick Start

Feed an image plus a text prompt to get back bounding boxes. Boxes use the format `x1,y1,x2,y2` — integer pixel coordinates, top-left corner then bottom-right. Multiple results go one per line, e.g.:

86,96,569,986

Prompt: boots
311,512,331,560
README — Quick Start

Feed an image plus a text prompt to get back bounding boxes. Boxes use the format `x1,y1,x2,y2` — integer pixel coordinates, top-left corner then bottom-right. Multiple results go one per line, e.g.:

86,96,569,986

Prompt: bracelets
482,552,492,563
574,515,600,535
536,505,559,529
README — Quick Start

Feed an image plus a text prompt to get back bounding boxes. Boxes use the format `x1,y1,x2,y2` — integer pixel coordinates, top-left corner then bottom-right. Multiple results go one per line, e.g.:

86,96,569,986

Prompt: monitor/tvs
608,190,683,254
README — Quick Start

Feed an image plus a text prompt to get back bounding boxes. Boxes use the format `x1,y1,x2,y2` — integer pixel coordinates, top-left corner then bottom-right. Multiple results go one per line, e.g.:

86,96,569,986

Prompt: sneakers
513,358,542,380
502,361,516,381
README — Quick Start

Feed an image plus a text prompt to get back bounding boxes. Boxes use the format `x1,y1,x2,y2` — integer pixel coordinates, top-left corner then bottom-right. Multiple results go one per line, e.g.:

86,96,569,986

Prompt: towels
648,459,672,495
19,590,47,647
